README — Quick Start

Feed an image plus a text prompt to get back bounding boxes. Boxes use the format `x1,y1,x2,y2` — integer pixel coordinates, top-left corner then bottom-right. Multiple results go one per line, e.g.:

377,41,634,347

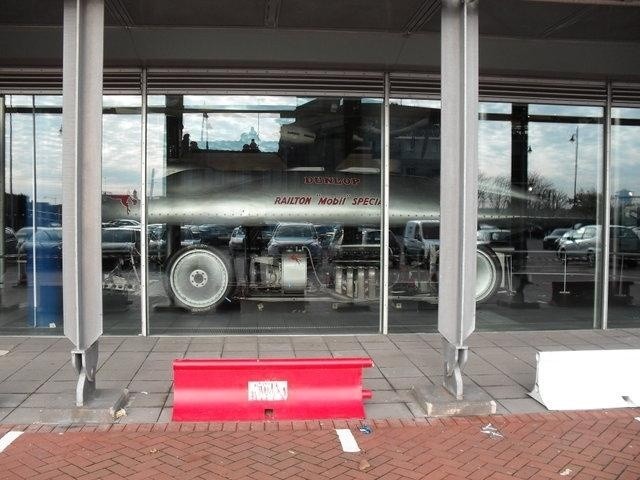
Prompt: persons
242,220,269,285
336,225,360,291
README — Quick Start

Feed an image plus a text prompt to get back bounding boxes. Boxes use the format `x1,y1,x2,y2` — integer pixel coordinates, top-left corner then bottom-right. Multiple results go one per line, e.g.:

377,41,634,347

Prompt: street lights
569,125,578,228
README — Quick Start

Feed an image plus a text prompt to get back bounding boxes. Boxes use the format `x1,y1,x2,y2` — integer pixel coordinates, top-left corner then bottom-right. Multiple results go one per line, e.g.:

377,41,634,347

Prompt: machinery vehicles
162,222,505,313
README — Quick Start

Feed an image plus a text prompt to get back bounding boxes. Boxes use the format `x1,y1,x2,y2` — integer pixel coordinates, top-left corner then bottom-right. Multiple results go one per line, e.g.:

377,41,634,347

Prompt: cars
3,220,502,273
556,225,640,265
542,228,569,250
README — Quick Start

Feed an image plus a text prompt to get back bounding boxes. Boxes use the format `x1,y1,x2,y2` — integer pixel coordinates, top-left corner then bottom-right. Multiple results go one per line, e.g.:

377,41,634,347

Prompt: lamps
527,145,533,155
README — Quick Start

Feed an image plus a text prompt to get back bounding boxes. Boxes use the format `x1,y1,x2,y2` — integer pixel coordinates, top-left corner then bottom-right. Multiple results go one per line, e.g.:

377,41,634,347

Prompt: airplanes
5,89,622,230
